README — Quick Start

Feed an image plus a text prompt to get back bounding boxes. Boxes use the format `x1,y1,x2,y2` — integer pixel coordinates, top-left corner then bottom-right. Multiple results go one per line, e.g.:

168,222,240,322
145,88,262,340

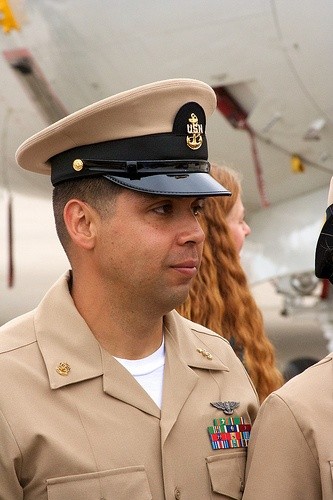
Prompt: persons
245,176,333,500
1,77,262,500
173,163,285,408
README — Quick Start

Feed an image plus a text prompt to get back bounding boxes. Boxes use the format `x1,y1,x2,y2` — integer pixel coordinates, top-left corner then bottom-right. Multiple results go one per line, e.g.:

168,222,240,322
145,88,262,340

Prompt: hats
15,79,232,198
315,177,333,279
